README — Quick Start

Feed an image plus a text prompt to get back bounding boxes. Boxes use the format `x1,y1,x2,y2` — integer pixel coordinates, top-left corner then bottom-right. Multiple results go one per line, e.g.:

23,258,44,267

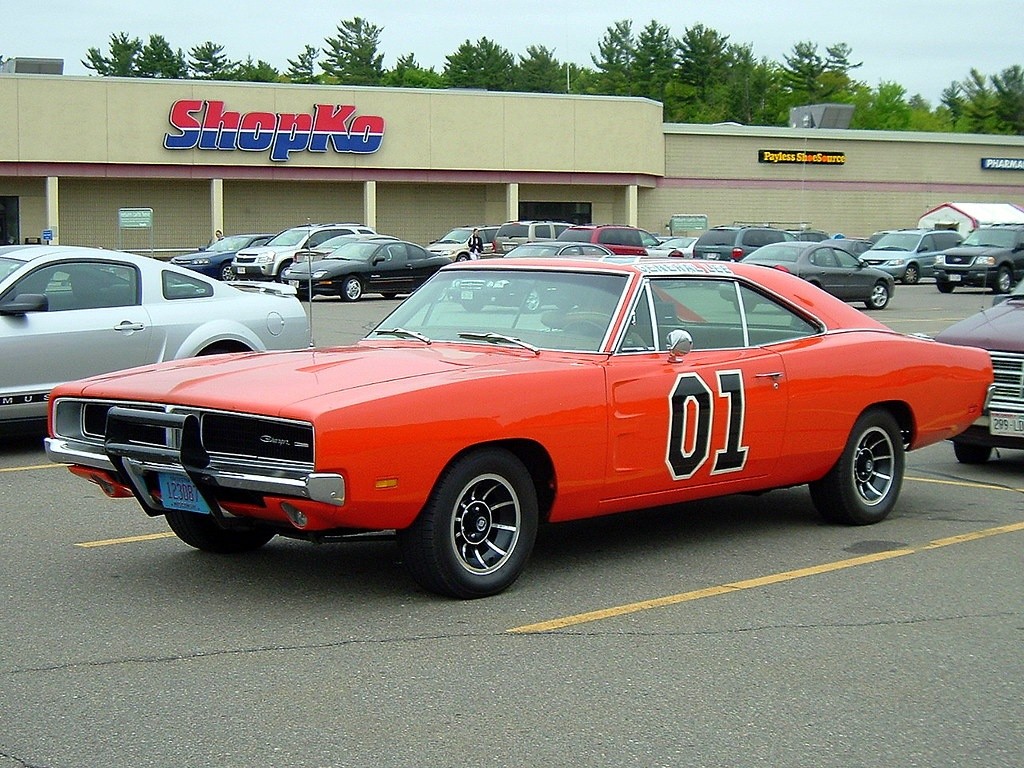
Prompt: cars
44,254,997,606
294,234,400,269
0,242,313,437
170,233,277,282
642,235,699,257
809,238,875,267
281,237,456,304
717,241,897,315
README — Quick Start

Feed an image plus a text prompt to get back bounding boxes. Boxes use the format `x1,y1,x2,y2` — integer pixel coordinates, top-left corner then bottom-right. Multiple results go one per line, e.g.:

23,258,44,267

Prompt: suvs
555,225,665,259
232,221,377,284
451,239,615,313
693,225,802,288
933,222,1024,294
426,223,501,263
856,228,964,283
933,263,1024,465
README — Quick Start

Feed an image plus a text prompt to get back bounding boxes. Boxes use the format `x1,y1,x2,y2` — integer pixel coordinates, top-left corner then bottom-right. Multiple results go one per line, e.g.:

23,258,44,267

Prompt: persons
215,230,226,242
466,228,484,261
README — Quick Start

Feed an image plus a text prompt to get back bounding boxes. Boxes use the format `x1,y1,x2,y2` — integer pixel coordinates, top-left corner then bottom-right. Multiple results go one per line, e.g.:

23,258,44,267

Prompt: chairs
815,256,828,266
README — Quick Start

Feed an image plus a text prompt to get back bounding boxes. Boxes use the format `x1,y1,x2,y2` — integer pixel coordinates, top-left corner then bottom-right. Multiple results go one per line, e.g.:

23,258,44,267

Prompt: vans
492,221,577,255
